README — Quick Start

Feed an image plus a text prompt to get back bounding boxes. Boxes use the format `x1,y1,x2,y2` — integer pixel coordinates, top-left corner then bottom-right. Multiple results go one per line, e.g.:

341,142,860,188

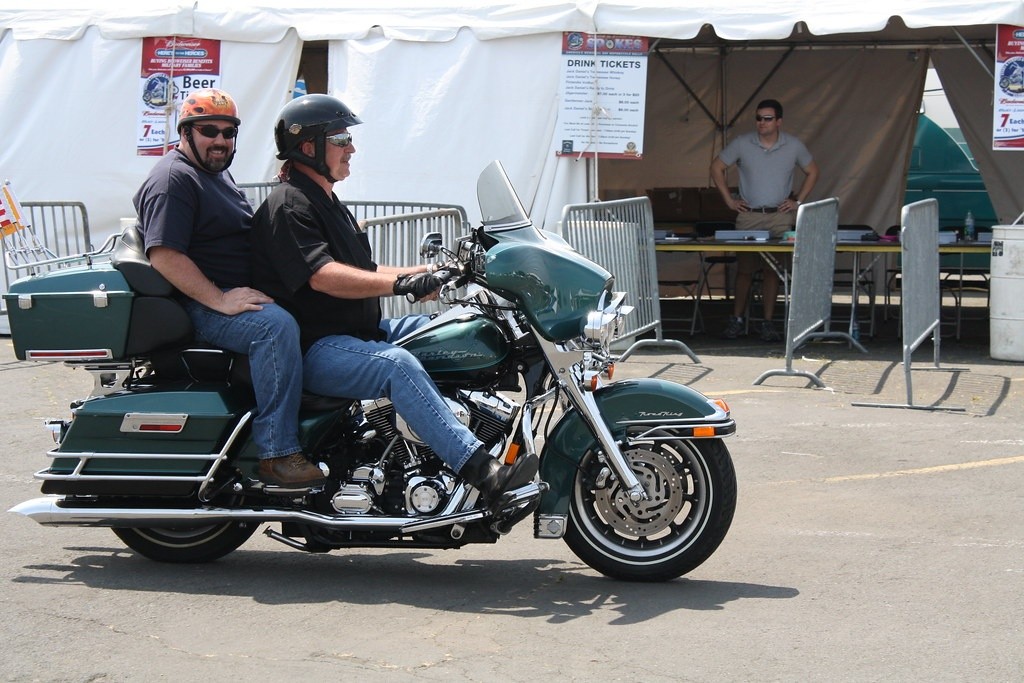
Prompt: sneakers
760,320,781,341
722,315,745,338
258,452,325,489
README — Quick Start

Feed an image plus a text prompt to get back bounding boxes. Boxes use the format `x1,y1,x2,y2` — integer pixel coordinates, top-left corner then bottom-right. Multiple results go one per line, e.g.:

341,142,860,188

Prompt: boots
458,445,539,516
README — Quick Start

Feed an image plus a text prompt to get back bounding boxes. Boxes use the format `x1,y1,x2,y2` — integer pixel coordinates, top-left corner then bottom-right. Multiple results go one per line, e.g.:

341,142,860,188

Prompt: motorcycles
2,160,738,582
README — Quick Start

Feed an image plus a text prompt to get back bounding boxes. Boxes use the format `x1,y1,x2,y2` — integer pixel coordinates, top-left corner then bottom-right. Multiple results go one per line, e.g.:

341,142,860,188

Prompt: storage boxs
3,265,133,364
47,389,231,474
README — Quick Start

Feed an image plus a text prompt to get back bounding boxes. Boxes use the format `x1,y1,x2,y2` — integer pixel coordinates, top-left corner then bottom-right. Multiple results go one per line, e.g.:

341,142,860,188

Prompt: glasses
755,114,775,122
188,122,237,139
326,133,352,147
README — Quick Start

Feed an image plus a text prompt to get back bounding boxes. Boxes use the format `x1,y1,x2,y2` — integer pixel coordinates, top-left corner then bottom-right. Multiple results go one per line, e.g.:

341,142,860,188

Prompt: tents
0,0,1024,338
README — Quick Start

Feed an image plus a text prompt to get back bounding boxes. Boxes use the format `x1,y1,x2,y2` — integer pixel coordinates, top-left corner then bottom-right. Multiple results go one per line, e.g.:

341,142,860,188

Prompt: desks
651,237,992,342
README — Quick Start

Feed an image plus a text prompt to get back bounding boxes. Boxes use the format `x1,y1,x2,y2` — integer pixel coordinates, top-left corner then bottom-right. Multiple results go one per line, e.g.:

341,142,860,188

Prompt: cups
965,213,975,240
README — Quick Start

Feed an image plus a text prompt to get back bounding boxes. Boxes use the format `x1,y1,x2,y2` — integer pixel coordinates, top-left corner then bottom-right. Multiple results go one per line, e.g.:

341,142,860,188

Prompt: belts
742,207,780,214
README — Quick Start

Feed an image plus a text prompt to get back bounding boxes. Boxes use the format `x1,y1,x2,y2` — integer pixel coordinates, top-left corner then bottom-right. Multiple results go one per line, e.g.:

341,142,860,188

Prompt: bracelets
427,264,434,274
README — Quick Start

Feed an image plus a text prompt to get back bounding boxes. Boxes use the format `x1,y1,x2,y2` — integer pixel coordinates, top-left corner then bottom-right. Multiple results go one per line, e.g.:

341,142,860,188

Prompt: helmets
177,88,241,135
274,93,363,160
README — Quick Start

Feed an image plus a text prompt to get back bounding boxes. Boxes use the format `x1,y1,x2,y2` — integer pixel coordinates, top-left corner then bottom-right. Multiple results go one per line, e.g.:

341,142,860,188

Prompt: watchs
795,200,801,205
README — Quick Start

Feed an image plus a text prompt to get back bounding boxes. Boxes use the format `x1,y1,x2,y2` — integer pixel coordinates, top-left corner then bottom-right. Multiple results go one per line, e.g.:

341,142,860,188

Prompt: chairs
652,222,994,340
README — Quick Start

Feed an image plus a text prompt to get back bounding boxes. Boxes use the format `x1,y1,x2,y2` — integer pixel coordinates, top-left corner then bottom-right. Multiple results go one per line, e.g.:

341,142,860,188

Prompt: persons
132,87,328,489
710,99,820,341
242,93,538,505
